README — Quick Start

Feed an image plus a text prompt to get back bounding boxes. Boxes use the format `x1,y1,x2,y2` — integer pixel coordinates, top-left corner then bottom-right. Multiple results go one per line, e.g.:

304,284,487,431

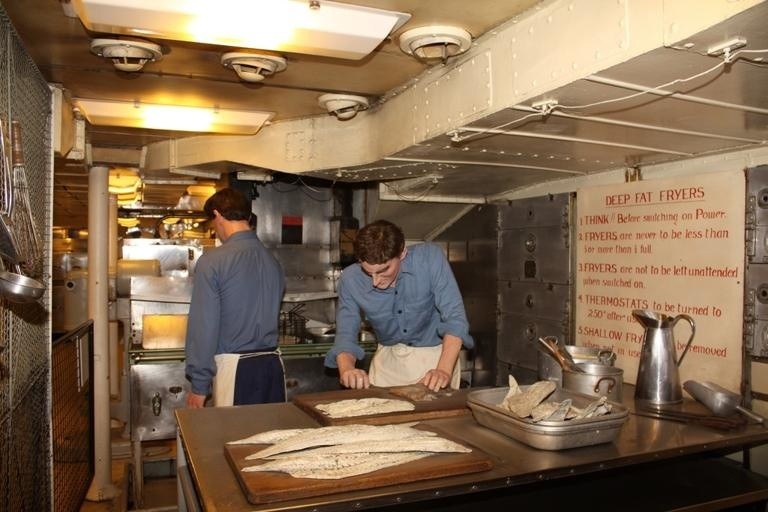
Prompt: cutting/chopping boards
291,384,474,427
224,423,495,504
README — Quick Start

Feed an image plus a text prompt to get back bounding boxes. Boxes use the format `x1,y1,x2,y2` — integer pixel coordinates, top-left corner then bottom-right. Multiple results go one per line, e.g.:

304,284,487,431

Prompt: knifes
630,406,746,432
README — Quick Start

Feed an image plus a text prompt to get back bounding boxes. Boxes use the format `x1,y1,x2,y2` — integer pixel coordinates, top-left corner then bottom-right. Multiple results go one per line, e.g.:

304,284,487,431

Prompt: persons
180,185,289,410
320,217,476,395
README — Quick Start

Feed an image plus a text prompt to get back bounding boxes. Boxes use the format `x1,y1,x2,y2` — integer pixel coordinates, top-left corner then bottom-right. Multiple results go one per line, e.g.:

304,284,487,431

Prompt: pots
532,335,624,402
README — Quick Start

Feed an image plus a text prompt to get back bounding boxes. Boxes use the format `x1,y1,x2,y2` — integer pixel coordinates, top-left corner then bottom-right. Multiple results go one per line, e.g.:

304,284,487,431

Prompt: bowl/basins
0,270,46,306
306,326,335,342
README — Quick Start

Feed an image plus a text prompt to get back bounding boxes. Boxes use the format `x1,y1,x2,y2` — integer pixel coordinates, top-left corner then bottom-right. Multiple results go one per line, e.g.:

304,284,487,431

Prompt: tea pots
632,310,694,403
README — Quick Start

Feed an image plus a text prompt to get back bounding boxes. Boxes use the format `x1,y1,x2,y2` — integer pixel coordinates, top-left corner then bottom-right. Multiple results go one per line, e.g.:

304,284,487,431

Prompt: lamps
59,0,412,61
69,97,277,135
